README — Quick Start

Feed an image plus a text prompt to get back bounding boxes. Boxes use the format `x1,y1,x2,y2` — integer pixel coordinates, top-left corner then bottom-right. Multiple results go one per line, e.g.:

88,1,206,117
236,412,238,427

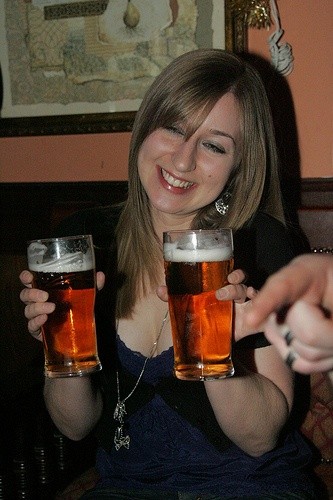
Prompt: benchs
0,178,333,500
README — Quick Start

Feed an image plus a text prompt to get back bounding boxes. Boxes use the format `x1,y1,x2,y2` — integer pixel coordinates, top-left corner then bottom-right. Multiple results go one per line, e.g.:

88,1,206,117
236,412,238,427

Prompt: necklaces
110,274,170,452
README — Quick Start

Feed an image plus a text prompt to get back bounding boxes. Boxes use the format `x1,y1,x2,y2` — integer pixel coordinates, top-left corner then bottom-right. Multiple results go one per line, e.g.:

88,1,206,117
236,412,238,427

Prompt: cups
163,228,234,381
26,236,102,378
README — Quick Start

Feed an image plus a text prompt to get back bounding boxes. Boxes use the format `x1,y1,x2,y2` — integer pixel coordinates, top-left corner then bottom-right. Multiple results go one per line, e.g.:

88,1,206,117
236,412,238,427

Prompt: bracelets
310,238,333,257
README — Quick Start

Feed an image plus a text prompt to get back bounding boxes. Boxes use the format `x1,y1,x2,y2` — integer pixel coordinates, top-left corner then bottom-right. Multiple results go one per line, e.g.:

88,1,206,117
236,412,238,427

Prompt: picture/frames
0,0,248,138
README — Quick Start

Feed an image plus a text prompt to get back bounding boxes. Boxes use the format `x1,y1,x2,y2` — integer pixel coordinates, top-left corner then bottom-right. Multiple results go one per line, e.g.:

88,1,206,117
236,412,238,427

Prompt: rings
280,350,298,368
281,328,297,347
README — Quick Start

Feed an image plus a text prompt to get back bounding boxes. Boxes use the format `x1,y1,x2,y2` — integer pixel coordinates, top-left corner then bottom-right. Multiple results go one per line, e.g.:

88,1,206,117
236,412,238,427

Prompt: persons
17,50,330,500
240,241,333,377
